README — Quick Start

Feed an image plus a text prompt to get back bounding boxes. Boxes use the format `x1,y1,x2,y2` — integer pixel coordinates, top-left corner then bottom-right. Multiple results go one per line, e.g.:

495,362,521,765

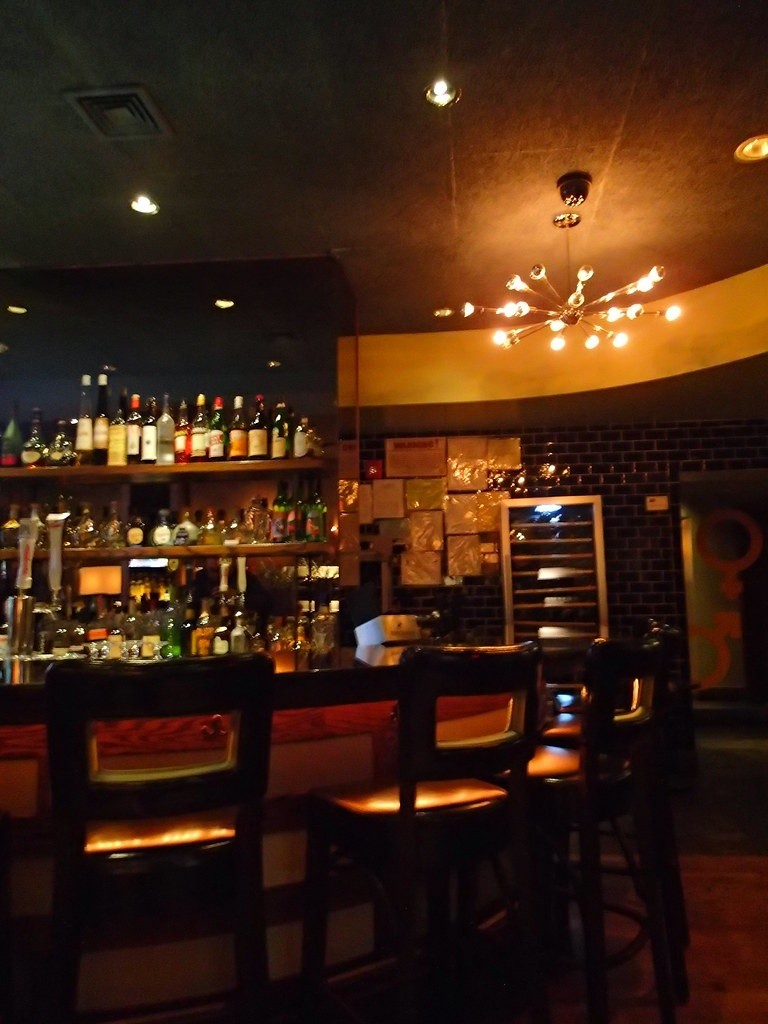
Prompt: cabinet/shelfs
0,458,335,559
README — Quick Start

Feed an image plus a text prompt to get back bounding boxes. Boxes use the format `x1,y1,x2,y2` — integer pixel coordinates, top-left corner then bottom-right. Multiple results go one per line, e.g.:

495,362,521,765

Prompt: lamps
460,213,684,353
422,62,463,111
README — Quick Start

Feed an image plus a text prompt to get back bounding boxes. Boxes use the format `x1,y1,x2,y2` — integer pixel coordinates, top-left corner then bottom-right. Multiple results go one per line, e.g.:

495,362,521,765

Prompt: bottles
418,610,441,640
123,506,147,548
96,500,126,548
0,494,95,550
271,478,328,543
74,372,175,465
20,407,45,468
42,418,78,466
175,393,269,463
0,399,23,468
146,497,271,547
271,401,324,459
1,556,338,662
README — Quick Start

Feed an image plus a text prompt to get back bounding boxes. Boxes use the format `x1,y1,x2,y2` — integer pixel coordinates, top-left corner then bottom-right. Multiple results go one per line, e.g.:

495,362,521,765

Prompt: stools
38,653,274,1024
455,619,687,1024
302,640,549,1024
